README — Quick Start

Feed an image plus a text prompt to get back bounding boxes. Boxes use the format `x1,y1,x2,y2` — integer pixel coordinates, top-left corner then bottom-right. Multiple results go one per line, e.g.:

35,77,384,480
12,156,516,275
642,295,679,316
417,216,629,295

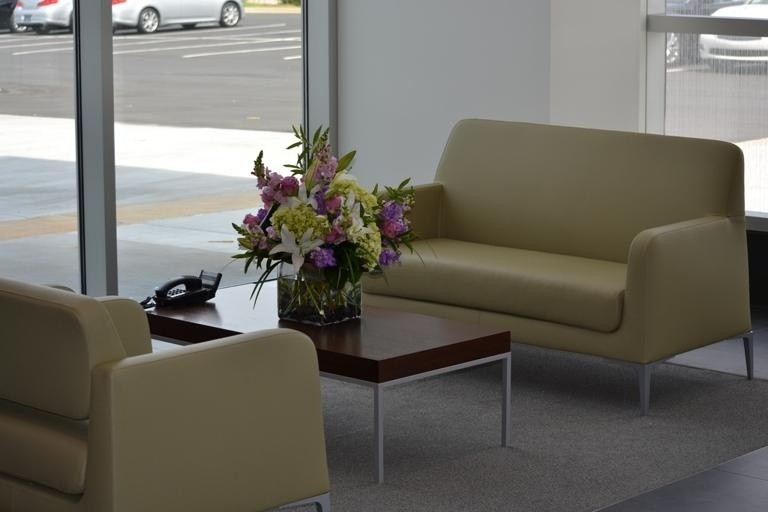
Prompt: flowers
222,125,439,317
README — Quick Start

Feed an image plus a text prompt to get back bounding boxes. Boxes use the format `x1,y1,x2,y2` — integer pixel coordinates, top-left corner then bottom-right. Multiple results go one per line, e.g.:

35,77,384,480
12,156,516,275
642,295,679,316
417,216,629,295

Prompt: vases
276,261,370,328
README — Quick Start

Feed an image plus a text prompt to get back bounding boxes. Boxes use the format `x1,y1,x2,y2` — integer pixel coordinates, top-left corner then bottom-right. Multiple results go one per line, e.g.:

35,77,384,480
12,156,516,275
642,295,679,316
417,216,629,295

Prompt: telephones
153,270,222,308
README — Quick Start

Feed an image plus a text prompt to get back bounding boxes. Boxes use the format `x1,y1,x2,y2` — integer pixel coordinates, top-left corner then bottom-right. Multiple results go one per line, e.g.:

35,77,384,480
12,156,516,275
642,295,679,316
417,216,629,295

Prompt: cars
1,0,246,34
666,0,768,69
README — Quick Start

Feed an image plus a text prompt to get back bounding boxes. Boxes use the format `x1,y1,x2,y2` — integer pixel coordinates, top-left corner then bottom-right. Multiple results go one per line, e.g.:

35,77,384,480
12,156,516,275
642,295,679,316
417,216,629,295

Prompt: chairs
0,277,332,510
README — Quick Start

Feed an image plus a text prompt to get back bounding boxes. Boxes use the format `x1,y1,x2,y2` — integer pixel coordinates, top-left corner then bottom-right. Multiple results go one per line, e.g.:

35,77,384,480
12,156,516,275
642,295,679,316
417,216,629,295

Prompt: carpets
280,342,766,510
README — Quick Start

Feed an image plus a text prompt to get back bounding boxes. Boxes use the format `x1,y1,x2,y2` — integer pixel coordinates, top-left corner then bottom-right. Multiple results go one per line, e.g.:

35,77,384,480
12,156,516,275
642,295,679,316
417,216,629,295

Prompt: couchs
363,116,756,417
